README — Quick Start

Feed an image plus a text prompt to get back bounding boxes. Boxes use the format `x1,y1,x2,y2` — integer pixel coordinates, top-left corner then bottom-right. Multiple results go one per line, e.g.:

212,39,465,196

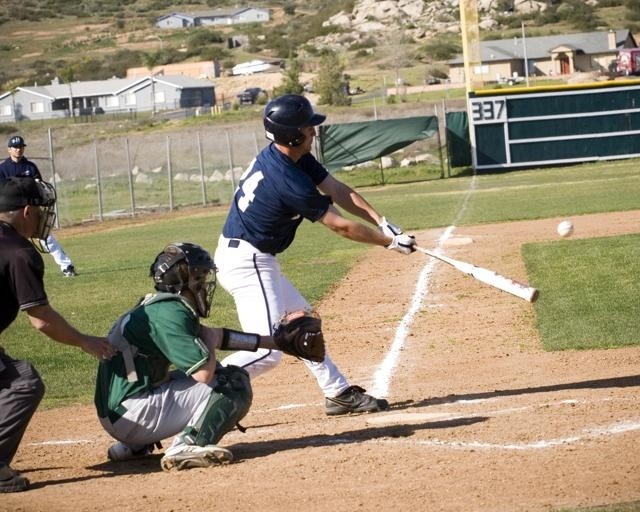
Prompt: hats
8,136,26,147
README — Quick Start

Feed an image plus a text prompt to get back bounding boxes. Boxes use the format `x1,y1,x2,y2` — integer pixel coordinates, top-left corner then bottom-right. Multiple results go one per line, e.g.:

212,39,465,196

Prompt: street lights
520,20,534,86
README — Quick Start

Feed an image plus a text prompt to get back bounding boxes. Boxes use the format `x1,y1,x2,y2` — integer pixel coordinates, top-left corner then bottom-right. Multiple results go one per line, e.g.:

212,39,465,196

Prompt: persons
91,240,324,476
0,133,78,281
217,92,417,417
0,174,120,496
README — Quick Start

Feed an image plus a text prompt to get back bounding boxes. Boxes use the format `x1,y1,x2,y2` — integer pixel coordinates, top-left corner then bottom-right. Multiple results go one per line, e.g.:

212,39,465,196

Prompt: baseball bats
413,245,538,302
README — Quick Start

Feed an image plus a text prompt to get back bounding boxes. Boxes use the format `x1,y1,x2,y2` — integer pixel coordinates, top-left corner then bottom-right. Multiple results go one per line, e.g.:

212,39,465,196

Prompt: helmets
0,175,57,253
149,242,219,318
264,94,326,147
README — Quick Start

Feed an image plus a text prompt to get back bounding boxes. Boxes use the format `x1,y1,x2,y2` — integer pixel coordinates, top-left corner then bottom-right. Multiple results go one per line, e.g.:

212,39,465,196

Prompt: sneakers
160,441,234,472
108,441,148,463
63,265,80,277
325,386,389,415
0,463,30,493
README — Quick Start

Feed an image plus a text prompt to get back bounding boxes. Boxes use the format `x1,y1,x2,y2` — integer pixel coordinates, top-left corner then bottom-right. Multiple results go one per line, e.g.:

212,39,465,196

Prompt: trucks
616,48,640,76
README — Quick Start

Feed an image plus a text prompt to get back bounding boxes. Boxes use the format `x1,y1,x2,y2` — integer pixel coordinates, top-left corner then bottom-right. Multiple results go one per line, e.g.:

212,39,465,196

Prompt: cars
350,87,364,95
428,79,442,85
239,87,268,105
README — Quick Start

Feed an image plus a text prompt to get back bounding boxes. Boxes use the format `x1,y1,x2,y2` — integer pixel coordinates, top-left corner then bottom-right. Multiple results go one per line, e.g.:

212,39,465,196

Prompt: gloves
376,216,417,255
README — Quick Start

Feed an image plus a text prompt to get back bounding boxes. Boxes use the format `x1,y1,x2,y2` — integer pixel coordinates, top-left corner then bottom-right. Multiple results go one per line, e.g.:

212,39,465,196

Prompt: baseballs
556,221,574,236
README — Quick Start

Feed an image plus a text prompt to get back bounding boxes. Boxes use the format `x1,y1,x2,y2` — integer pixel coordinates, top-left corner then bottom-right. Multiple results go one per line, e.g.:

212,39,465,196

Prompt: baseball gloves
274,309,325,361
